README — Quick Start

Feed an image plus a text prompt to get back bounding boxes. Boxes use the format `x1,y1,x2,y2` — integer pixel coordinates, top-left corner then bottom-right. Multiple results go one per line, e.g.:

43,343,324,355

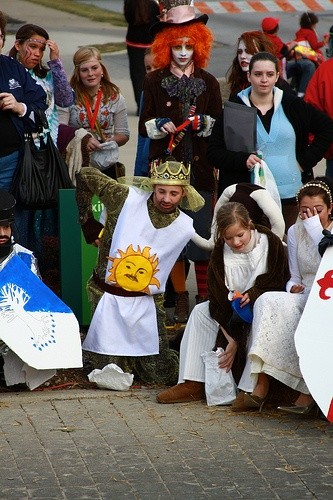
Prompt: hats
150,5,209,41
0,191,17,221
261,16,280,32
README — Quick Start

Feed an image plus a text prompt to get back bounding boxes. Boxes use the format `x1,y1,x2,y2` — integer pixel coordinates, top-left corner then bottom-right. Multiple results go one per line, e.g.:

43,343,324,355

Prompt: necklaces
84,82,102,100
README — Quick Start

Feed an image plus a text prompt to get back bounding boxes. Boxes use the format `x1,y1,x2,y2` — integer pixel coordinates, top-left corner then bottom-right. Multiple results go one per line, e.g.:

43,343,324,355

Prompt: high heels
232,392,264,412
277,403,318,417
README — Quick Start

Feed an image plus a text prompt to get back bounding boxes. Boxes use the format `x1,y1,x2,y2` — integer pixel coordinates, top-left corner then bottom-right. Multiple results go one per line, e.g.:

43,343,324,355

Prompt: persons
156,202,289,412
0,0,333,417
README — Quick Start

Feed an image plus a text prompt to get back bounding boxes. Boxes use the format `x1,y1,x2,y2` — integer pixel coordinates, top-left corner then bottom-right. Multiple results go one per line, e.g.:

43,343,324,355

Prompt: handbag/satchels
11,134,73,207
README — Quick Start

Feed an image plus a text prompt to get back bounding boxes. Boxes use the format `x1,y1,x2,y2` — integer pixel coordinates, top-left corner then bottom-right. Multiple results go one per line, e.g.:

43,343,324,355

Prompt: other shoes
297,92,305,97
156,382,204,402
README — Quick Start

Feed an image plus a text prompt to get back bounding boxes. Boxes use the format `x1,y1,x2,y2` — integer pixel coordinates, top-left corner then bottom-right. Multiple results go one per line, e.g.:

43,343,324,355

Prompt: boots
196,295,209,306
173,291,188,323
